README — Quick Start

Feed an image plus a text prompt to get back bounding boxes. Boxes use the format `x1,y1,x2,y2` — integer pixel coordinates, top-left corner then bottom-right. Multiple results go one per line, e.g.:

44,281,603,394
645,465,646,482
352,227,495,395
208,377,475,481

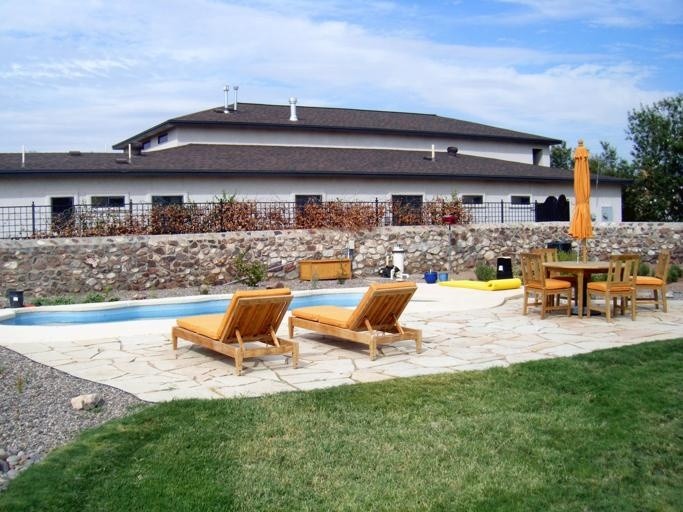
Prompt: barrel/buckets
9,290,23,308
438,273,448,282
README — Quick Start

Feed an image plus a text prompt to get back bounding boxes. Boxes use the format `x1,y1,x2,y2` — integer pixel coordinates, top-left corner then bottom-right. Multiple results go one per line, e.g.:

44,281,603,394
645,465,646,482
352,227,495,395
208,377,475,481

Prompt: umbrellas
568,138,593,264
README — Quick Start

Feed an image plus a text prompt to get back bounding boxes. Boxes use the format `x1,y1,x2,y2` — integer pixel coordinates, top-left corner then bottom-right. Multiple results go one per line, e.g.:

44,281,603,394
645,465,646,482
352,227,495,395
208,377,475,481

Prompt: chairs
172,287,300,377
287,280,423,360
519,247,672,323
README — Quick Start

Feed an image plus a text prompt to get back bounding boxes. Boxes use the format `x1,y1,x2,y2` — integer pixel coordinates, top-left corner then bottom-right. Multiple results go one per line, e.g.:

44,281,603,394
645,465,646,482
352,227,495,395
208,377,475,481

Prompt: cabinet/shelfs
297,259,353,281
